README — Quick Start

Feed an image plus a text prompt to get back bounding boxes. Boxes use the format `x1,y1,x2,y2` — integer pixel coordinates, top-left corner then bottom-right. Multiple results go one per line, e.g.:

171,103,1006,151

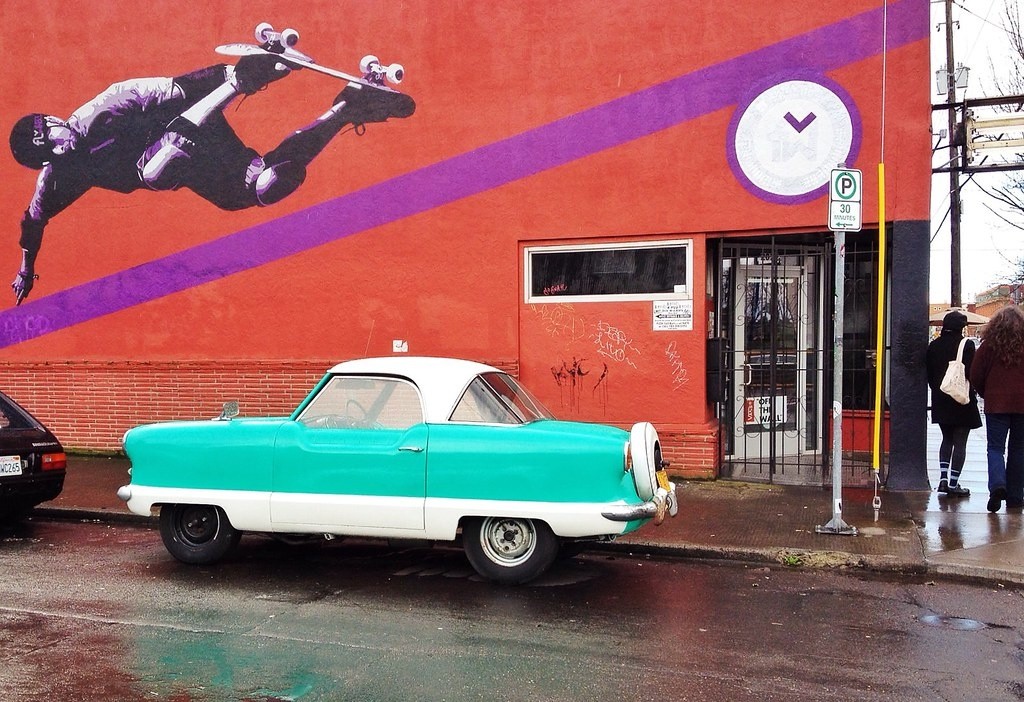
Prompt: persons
970,304,1024,510
928,310,984,498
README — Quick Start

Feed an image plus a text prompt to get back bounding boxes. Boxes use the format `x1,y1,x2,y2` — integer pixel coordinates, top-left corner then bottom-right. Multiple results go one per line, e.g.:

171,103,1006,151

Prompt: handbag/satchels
940,337,970,405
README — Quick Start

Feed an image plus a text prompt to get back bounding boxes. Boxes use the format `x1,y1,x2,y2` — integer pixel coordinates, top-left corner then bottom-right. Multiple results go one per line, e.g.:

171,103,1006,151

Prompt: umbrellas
929,306,991,326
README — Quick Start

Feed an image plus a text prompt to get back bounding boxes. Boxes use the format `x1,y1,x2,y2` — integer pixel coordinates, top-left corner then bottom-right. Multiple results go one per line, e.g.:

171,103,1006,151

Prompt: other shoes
987,487,1007,512
1006,499,1024,507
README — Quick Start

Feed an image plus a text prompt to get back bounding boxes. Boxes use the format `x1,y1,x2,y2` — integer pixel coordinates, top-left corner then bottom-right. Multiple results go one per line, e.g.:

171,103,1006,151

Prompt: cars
117,355,679,586
0,391,67,514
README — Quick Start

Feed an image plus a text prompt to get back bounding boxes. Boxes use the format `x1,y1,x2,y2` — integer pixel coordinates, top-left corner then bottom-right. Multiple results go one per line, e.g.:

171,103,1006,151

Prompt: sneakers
938,481,948,492
948,483,970,497
